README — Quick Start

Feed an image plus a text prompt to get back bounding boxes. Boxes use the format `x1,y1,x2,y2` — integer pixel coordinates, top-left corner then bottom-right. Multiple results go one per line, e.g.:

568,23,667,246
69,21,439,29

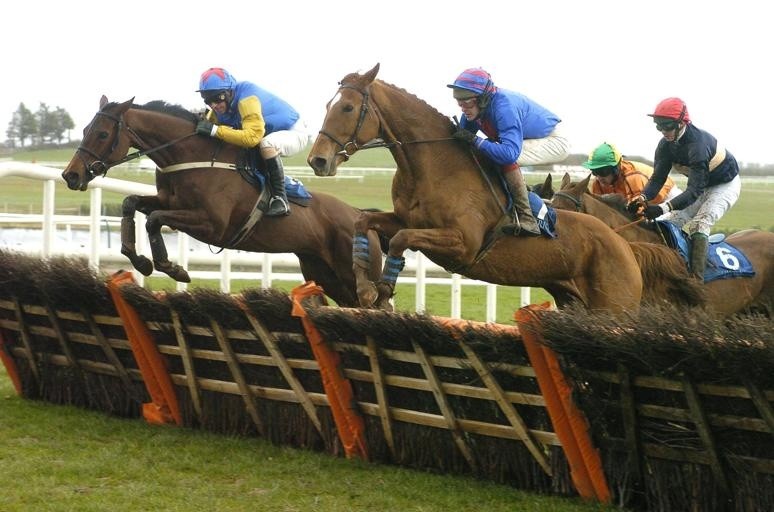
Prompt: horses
307,63,642,317
526,175,555,201
546,172,774,323
61,96,393,312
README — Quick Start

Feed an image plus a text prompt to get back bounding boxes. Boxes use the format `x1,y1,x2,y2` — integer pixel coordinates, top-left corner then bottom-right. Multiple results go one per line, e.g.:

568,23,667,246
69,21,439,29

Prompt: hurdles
115,271,359,464
531,303,769,510
307,282,594,503
0,251,170,426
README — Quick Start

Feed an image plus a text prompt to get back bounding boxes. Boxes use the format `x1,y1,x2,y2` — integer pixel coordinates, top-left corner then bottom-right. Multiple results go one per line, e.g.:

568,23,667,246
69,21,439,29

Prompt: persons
197,66,311,217
585,141,685,220
448,66,573,235
629,96,743,291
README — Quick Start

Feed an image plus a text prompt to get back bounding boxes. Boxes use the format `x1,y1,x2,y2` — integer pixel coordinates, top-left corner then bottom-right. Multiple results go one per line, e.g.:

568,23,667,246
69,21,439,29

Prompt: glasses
457,99,478,108
590,166,617,176
656,121,680,132
204,92,229,104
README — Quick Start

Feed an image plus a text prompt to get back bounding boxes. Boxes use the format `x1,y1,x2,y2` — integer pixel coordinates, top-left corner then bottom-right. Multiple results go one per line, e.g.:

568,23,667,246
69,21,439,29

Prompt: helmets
582,141,622,186
446,68,495,121
196,68,236,114
648,98,690,146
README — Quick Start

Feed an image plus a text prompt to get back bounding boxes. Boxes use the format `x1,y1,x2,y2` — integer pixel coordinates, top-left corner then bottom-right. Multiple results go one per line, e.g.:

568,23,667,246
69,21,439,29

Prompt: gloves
452,116,477,145
644,201,672,220
626,194,646,213
196,116,212,136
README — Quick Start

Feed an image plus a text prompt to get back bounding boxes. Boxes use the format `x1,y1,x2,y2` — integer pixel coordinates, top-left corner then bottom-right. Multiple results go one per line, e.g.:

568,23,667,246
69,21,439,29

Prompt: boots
266,155,291,216
502,164,541,236
691,233,709,285
